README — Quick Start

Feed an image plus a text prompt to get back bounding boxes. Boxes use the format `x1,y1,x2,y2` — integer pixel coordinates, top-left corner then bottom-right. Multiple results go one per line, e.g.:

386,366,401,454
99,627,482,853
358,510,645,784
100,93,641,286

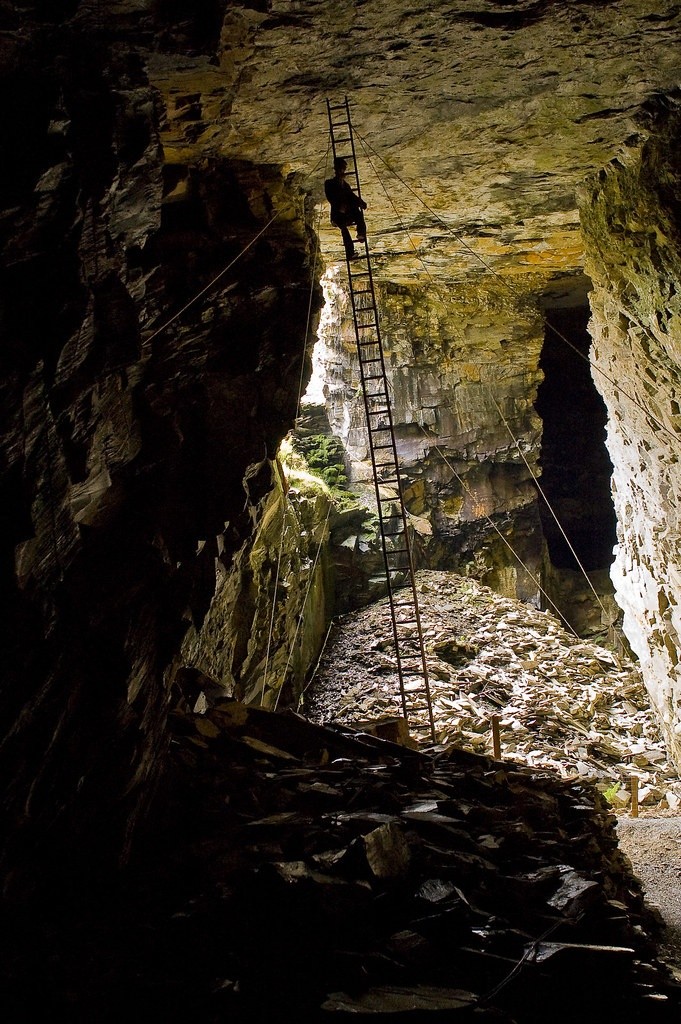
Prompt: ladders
323,90,439,757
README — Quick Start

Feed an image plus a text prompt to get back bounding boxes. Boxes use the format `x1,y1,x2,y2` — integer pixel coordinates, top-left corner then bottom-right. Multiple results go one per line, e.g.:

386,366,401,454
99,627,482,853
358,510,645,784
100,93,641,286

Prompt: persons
323,157,366,260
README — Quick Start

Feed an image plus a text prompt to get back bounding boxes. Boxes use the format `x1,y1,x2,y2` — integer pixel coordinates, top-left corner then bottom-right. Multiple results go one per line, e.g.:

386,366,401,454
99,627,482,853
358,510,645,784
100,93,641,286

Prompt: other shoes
351,253,359,259
358,236,364,243
